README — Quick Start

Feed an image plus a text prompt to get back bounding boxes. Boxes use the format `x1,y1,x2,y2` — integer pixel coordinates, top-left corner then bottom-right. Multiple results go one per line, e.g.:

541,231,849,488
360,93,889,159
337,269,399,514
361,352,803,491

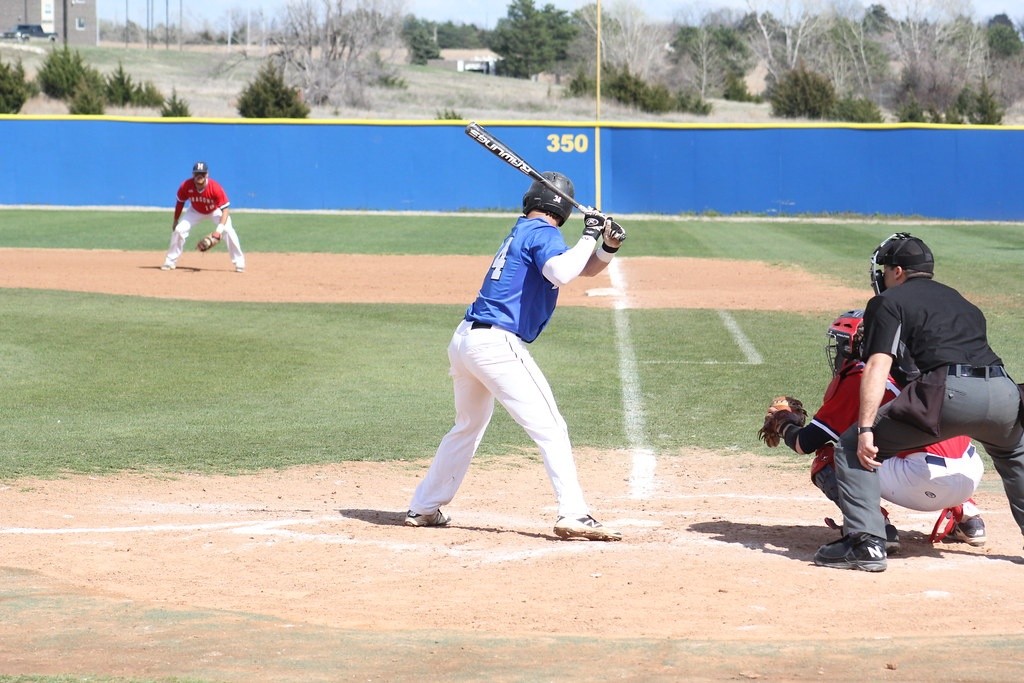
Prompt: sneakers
553,513,621,540
884,524,901,554
814,532,887,572
944,517,986,546
404,508,451,526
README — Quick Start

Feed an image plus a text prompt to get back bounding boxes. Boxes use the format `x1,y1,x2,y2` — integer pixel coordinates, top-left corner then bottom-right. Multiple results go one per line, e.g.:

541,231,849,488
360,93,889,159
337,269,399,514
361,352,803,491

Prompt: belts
926,446,975,467
949,365,1007,378
472,322,491,330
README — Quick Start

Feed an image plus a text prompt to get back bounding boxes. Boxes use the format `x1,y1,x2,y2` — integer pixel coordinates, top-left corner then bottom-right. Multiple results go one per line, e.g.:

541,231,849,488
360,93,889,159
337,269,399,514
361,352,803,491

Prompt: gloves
582,205,606,241
602,216,625,253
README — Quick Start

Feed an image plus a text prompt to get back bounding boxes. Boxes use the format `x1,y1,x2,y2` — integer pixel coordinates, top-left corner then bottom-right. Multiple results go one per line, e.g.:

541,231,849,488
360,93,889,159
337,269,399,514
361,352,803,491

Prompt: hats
192,162,207,173
876,236,934,272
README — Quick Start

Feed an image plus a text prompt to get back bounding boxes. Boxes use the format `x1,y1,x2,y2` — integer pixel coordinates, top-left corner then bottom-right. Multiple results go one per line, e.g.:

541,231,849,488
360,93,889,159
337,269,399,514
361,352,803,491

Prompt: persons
814,232,1024,573
161,161,245,273
757,310,987,556
404,121,627,542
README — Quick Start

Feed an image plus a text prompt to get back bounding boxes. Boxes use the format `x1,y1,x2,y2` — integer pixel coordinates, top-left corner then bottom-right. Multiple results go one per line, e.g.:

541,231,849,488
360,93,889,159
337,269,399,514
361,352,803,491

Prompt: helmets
523,171,575,226
829,309,865,359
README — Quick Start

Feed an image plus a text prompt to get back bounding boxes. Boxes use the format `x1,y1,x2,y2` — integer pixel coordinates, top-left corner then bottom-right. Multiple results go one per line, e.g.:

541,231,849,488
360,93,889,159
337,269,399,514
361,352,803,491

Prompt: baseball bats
464,122,627,242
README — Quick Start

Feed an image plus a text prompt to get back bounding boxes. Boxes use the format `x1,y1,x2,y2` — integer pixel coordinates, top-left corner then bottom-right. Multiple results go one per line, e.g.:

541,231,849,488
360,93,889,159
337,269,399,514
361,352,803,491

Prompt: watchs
858,426,874,435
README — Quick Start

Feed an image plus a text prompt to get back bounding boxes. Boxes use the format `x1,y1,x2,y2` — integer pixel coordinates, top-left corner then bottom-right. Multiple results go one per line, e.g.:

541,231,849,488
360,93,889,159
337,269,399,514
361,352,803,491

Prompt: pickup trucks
0,23,60,44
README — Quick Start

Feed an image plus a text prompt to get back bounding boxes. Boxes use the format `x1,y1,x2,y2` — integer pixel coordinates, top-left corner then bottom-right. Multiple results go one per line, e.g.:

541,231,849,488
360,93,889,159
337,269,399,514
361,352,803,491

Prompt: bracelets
216,223,225,234
596,246,615,263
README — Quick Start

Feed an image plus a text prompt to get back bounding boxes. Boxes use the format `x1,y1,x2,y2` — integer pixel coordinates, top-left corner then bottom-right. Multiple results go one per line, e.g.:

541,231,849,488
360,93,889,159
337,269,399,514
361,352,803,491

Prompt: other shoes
161,265,176,270
236,268,244,272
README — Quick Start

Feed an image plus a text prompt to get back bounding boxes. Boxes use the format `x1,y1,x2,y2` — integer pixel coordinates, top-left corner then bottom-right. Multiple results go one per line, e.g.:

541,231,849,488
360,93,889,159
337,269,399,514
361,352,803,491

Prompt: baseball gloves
197,232,222,251
759,395,808,447
